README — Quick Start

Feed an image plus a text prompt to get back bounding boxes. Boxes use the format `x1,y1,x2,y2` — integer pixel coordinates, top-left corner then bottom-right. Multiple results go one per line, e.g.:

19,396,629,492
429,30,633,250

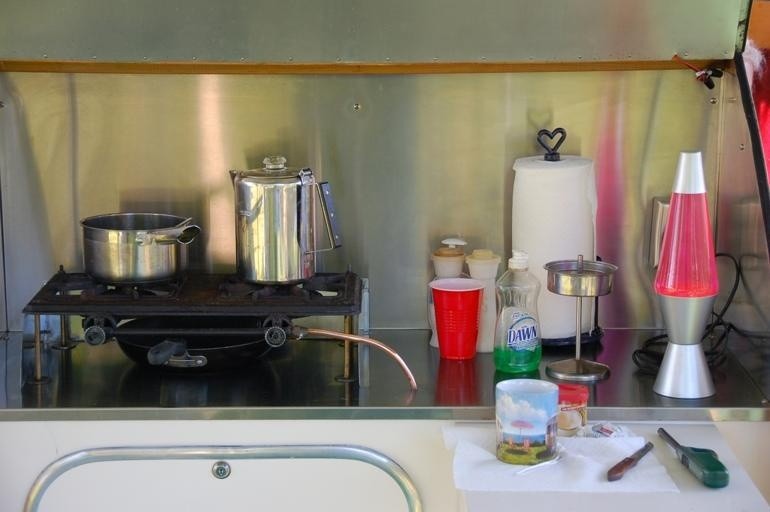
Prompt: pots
79,213,201,284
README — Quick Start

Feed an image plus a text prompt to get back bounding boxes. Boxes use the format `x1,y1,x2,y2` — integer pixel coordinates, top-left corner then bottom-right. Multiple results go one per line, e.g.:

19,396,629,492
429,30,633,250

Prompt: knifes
608,442,654,480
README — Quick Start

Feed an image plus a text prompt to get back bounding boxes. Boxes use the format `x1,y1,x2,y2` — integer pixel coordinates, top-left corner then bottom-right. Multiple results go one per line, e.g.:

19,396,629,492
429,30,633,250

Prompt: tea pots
229,156,342,286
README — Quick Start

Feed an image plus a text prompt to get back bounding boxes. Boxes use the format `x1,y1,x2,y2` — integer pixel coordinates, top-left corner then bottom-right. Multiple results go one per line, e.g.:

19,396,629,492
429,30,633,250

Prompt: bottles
466,248,500,353
426,248,467,349
557,384,587,436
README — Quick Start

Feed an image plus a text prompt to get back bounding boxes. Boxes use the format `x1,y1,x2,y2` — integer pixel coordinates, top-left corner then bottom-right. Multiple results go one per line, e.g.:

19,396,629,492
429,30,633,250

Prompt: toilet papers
512,154,599,342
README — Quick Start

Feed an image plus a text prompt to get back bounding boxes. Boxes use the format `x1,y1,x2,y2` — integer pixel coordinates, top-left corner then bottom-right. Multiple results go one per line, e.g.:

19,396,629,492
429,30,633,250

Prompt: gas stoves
22,266,362,347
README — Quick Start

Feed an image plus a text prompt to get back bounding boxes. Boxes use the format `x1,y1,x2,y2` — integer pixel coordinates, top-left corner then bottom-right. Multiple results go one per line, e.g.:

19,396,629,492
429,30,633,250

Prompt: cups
494,378,560,462
429,277,484,360
